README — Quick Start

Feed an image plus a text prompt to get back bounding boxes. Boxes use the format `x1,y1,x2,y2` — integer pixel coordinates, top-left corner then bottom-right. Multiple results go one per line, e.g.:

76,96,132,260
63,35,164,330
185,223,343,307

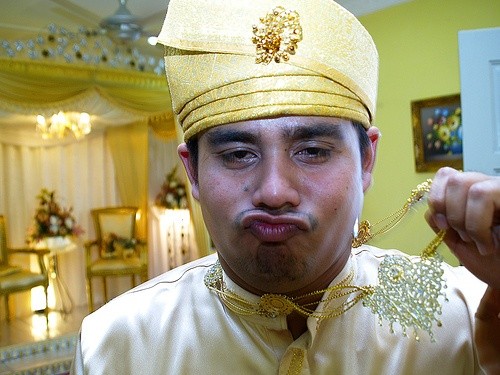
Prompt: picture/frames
411,93,464,174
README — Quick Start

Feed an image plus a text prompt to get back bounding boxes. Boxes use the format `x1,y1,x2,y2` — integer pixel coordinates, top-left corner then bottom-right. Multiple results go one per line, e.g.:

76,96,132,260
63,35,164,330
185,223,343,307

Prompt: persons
70,0,499,375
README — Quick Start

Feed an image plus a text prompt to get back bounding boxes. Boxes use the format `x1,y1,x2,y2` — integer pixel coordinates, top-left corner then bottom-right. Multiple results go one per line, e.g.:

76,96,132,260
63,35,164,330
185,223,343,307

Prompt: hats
158,0,380,141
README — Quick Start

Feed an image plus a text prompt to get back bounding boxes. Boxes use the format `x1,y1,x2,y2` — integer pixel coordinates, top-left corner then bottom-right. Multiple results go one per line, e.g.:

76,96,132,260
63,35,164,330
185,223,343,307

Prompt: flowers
28,188,84,240
104,232,142,258
154,166,188,210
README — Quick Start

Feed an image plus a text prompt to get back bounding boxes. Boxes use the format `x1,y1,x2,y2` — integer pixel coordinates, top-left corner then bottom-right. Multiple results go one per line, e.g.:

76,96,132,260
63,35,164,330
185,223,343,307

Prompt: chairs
85,206,147,311
0,215,50,326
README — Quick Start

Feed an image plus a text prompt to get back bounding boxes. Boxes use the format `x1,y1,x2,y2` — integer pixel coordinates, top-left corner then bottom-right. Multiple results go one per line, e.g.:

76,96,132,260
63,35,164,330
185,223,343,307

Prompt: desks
28,235,79,315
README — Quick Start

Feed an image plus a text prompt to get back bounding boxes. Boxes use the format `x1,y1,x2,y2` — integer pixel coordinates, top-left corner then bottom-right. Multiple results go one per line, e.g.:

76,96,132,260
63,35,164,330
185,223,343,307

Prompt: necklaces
202,176,450,343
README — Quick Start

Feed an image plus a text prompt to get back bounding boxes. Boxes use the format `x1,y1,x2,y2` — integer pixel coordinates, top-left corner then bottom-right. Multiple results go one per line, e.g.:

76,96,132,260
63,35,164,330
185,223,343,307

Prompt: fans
52,0,168,45
